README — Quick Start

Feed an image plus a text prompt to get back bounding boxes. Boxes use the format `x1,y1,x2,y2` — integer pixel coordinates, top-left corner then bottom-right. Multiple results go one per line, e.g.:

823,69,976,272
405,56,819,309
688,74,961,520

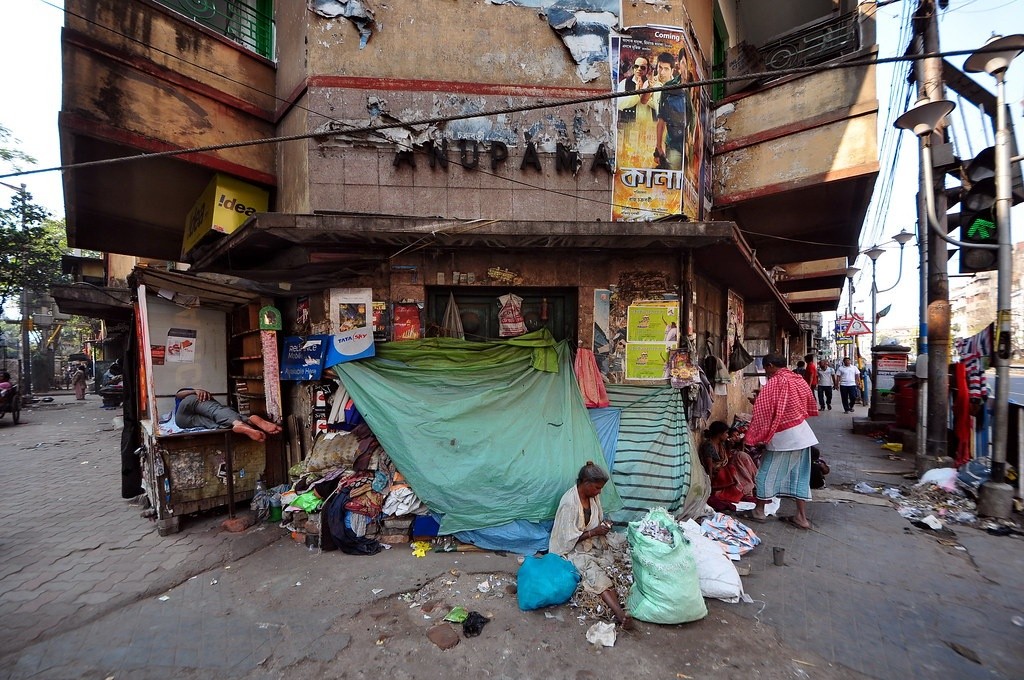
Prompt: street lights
893,31,1024,517
845,229,915,349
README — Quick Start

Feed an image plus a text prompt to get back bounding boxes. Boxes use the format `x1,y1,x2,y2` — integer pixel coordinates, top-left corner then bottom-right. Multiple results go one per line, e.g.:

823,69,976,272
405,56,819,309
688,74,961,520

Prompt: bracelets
589,531,591,537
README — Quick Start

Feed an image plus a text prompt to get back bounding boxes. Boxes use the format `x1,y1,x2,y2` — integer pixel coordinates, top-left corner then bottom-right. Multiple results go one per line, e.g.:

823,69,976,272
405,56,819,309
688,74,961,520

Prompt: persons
742,352,820,529
803,354,817,393
818,360,835,411
661,315,677,341
792,361,805,375
549,461,635,629
174,387,282,442
72,365,86,400
65,373,70,390
836,357,861,414
702,421,758,497
617,48,696,170
0,370,12,388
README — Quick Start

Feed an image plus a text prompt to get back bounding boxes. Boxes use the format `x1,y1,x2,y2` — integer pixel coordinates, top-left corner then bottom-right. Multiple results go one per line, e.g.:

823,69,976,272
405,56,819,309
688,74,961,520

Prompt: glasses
620,341,626,349
634,65,646,70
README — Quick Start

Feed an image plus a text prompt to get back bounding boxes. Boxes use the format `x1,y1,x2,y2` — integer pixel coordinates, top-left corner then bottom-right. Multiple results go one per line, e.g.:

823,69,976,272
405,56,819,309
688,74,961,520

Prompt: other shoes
845,410,849,414
820,405,825,411
827,403,832,411
850,408,854,412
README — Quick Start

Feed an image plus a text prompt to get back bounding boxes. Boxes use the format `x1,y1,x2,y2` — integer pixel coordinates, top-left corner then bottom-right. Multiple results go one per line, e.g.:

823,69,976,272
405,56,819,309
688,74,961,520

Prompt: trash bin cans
894,372,918,429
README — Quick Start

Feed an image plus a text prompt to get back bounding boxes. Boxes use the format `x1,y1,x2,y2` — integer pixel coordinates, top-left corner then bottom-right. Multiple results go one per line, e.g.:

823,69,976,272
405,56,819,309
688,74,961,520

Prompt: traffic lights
916,186,961,261
959,146,1000,273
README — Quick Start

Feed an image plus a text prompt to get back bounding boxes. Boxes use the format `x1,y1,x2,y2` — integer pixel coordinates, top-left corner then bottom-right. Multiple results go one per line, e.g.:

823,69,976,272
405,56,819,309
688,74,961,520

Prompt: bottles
254,482,265,495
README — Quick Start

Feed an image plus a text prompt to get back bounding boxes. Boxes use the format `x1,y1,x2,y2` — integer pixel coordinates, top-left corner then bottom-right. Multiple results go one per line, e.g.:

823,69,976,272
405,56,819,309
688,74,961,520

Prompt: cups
773,546,784,566
255,508,269,522
271,506,281,520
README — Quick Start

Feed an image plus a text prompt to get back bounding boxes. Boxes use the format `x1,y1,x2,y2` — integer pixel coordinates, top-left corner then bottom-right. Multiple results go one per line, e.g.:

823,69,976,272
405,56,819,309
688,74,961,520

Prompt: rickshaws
0,385,22,424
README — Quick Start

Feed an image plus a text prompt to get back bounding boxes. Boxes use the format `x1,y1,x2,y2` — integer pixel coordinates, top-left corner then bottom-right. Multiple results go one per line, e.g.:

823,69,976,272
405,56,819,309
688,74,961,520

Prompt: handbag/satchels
730,325,754,372
499,293,528,336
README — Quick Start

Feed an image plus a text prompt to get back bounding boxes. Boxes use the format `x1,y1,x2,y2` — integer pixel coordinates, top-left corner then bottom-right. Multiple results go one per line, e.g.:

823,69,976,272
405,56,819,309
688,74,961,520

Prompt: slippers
735,509,766,523
779,515,809,530
